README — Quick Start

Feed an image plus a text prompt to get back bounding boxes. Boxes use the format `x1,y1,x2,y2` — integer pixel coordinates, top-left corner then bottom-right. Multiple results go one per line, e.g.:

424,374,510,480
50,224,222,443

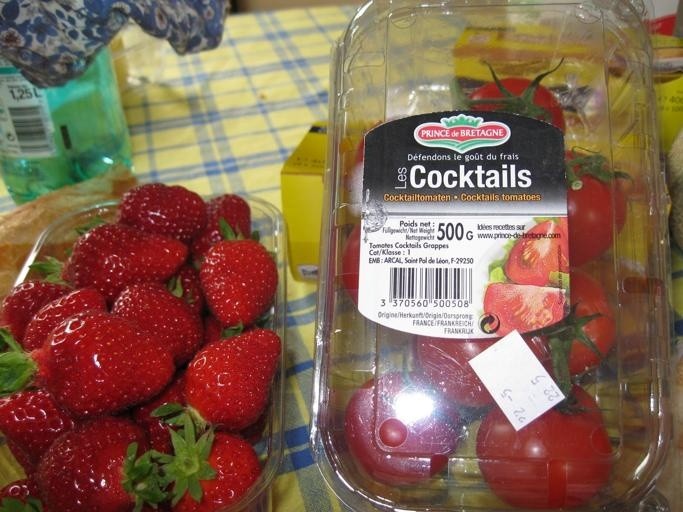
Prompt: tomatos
343,56,629,510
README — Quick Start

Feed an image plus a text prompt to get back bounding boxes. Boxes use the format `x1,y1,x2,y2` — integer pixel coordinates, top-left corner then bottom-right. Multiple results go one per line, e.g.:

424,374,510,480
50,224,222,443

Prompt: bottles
1,38,134,207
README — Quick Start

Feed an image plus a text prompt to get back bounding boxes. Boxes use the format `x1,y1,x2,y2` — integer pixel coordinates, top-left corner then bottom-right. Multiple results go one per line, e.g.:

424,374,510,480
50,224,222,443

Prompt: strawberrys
0,183,280,511
